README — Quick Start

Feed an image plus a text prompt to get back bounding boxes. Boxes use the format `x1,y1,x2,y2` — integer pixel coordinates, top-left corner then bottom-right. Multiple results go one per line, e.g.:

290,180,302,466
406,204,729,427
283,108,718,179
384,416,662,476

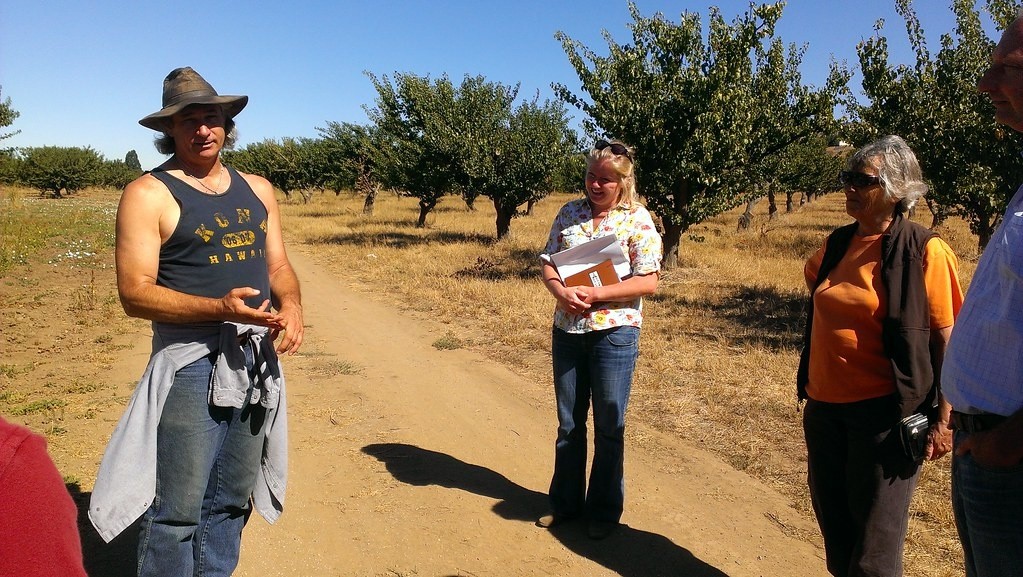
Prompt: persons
0,417,89,577
795,134,964,577
940,6,1023,577
85,66,304,577
536,140,664,540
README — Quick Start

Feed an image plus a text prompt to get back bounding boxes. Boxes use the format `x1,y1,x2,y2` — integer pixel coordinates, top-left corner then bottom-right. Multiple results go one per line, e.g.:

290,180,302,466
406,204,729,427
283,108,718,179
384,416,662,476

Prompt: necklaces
175,158,225,194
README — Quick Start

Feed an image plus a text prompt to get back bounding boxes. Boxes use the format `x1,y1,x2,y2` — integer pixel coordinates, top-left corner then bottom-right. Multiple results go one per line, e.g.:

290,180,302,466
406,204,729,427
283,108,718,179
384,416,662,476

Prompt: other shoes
588,514,617,538
536,504,582,527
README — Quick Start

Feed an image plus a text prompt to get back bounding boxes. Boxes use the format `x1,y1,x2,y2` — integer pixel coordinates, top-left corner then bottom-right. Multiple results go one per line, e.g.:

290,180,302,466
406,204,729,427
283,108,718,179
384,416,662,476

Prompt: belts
947,409,1023,432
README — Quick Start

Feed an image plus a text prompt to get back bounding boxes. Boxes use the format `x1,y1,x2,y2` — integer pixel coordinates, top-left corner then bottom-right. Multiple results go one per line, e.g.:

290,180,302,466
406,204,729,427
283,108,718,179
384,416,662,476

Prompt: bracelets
547,278,562,282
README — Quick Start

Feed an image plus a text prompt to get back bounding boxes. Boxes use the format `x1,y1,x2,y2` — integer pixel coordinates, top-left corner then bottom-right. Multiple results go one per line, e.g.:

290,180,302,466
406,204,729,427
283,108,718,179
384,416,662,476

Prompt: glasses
840,171,886,187
595,139,635,165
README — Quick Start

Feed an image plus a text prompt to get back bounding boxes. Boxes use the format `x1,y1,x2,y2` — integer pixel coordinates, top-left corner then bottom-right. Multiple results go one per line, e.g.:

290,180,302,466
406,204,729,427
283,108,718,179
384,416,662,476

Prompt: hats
138,66,248,132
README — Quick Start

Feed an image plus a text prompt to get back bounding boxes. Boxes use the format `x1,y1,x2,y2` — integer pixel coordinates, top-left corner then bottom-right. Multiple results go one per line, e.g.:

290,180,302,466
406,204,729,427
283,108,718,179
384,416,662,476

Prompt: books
564,259,623,287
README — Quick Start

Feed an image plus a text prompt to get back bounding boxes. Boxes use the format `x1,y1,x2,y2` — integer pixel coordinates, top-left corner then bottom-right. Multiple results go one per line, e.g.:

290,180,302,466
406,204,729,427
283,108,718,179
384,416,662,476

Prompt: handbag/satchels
900,404,944,462
